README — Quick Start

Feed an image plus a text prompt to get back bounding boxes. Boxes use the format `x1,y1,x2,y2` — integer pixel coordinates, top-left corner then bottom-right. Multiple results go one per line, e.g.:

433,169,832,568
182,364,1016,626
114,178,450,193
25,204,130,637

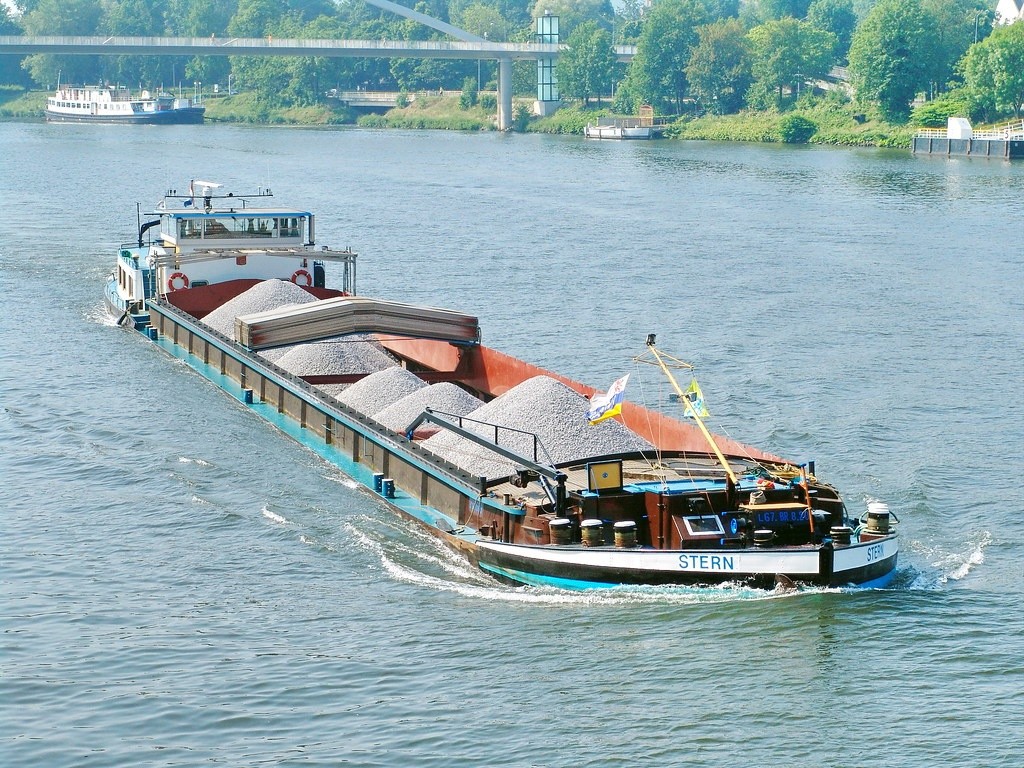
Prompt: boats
42,69,206,125
104,179,900,595
583,121,654,139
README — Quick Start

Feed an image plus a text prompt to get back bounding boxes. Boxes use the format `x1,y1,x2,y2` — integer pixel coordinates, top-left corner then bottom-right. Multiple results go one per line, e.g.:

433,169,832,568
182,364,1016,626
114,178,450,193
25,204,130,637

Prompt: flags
586,374,630,426
683,380,710,419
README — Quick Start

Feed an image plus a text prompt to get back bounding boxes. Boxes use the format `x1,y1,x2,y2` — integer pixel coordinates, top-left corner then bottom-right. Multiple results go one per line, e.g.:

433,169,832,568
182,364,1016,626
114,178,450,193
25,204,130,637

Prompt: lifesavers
168,273,189,292
291,270,312,288
